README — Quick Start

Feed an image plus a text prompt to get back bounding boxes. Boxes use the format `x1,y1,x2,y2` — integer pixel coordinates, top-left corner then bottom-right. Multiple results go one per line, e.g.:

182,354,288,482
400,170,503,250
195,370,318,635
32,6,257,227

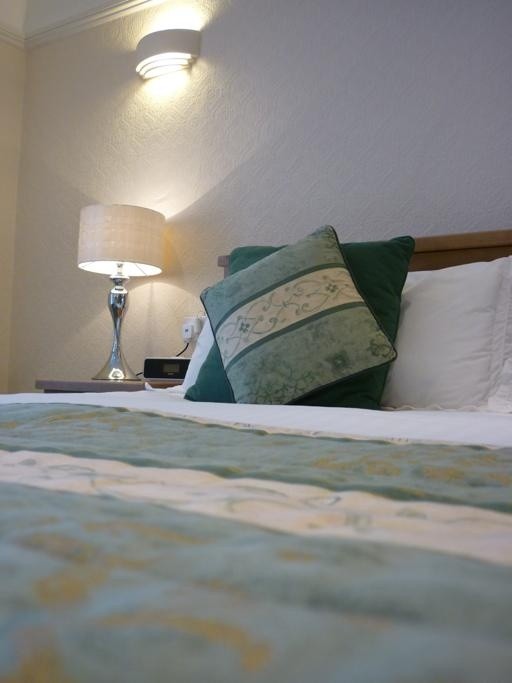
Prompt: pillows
174,252,512,416
184,232,421,412
199,227,400,412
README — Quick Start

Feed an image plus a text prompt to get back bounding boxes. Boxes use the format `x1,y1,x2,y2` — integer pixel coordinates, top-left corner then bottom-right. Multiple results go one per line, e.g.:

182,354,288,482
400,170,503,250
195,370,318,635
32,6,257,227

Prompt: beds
1,231,512,683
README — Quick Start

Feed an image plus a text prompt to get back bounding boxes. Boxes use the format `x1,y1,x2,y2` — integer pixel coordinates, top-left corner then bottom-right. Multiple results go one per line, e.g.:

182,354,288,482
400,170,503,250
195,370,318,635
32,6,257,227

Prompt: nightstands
36,379,184,394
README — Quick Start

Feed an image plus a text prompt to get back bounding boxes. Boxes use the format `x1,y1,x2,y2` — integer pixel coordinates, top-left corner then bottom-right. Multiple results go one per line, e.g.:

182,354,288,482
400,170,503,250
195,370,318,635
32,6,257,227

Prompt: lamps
135,29,201,80
77,204,165,381
